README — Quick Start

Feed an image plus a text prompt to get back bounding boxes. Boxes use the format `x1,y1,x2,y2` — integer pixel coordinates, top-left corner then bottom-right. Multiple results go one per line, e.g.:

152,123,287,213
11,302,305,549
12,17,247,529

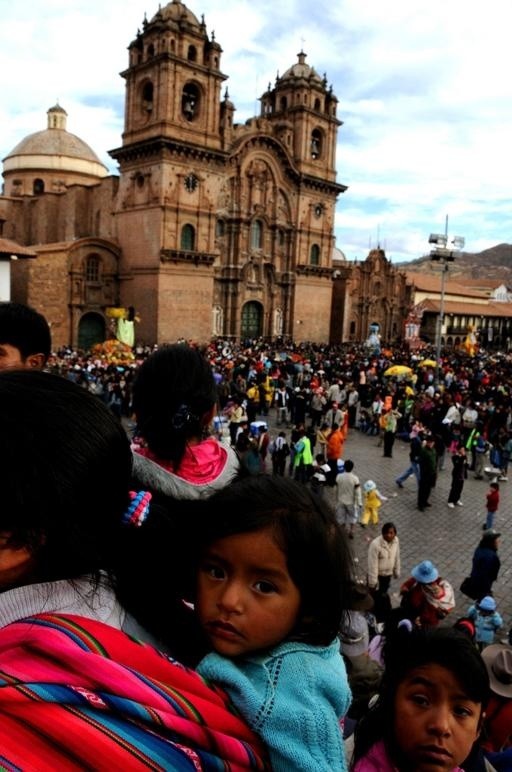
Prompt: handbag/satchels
460,577,480,601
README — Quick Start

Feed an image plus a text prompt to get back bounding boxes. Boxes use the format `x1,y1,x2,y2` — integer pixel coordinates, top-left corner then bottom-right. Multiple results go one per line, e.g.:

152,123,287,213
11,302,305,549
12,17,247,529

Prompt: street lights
428,214,465,392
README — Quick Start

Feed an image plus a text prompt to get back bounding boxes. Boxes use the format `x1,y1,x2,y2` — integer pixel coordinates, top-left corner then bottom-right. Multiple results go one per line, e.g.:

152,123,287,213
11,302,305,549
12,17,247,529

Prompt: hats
348,584,374,611
479,596,496,611
364,480,376,491
481,644,512,698
483,528,501,539
411,560,439,583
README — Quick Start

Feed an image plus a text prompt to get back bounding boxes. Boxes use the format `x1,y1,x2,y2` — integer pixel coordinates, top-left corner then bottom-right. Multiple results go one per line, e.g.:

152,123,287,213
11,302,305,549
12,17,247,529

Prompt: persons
349,624,512,771
192,469,352,769
476,640,510,772
333,607,384,771
1,301,53,376
177,335,511,539
39,335,158,439
337,523,511,673
0,368,274,772
